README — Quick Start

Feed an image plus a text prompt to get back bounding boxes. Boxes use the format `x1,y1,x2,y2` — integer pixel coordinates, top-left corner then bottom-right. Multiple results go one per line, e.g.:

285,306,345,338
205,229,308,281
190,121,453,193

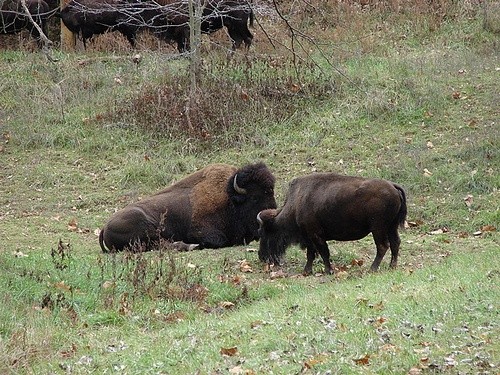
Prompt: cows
257,173,407,276
99,161,277,253
39,0,141,52
128,0,254,53
0,0,49,49
